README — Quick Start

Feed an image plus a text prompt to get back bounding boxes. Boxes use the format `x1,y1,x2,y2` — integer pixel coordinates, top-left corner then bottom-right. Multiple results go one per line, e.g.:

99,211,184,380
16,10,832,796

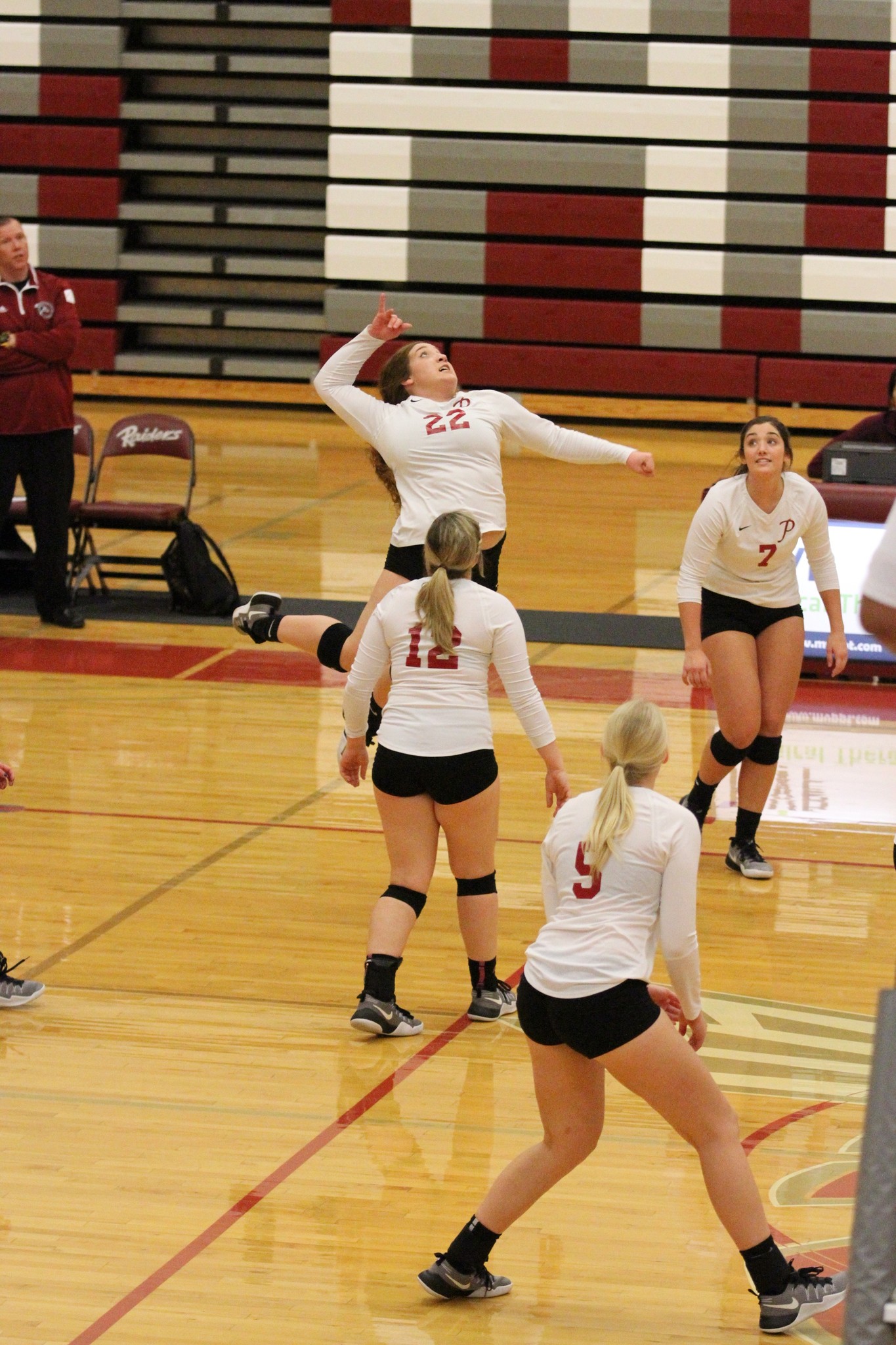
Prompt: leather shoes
41,604,85,628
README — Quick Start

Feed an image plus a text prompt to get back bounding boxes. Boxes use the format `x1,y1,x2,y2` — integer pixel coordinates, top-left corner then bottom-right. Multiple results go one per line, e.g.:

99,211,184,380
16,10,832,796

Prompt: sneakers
350,987,423,1036
467,980,517,1021
0,951,46,1007
232,591,282,644
679,794,709,834
725,837,774,880
417,1252,513,1299
748,1258,850,1334
337,706,379,767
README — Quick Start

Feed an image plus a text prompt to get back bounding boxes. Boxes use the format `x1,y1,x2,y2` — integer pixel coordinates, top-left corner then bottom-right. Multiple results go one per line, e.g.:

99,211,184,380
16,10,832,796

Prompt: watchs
0,330,9,349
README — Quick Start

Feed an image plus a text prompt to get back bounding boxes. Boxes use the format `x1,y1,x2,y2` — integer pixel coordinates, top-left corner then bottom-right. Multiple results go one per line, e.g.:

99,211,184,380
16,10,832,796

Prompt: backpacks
162,519,240,617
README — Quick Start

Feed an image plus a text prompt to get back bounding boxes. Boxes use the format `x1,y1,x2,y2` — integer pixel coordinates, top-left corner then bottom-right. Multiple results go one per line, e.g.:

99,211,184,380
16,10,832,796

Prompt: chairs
65,412,197,606
10,413,100,600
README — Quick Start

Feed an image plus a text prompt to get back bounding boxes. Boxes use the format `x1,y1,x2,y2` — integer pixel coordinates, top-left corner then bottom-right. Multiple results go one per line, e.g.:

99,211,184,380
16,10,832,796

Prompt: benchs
0,0,896,428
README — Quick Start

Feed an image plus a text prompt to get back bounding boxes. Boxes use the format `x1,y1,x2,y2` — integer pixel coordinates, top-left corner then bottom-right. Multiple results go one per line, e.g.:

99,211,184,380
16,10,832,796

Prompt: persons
1,209,95,629
414,411,896,1334
232,291,654,750
336,507,575,1041
0,763,46,1010
